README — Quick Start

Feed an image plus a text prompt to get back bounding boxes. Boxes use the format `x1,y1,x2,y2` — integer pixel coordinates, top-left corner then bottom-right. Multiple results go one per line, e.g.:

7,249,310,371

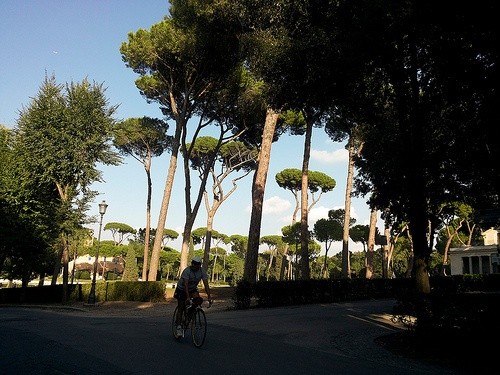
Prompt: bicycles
170,294,214,347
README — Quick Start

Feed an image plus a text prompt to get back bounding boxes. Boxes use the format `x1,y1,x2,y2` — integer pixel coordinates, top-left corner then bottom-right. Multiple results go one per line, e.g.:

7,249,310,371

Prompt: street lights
84,200,109,305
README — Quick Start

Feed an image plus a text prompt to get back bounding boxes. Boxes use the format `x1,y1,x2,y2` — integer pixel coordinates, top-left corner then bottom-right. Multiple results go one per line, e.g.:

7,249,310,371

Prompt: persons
174,257,214,337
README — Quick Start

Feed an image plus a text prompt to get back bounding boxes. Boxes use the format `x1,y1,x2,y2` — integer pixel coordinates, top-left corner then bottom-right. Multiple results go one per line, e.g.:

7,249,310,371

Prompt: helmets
192,257,202,264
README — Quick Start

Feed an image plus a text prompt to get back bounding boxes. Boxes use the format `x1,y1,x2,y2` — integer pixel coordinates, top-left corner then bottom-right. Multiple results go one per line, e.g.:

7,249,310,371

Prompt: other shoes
176,325,184,335
